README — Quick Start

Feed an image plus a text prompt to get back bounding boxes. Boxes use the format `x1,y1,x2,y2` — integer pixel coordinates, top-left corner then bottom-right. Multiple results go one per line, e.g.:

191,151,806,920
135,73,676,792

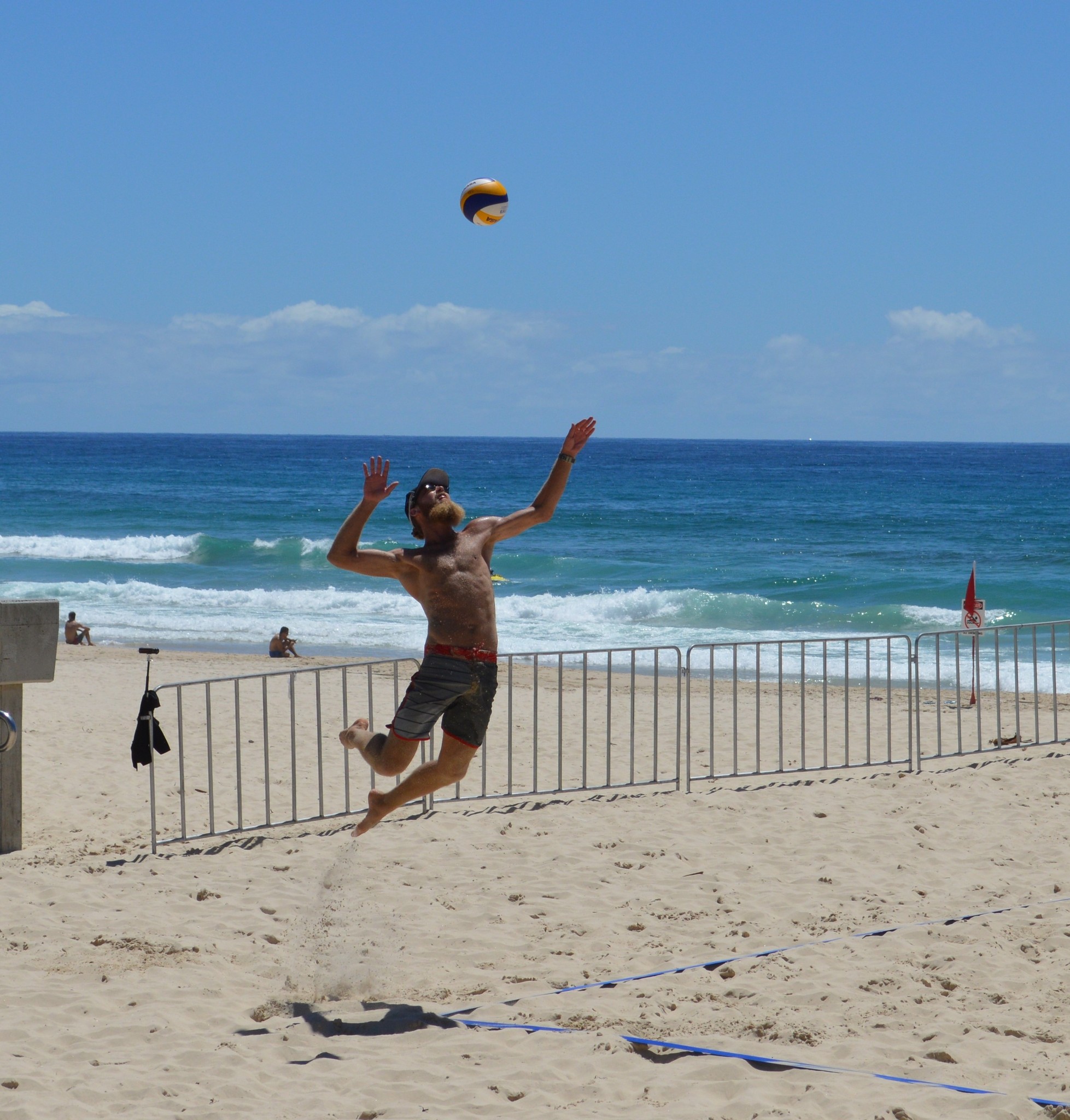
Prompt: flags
963,565,975,616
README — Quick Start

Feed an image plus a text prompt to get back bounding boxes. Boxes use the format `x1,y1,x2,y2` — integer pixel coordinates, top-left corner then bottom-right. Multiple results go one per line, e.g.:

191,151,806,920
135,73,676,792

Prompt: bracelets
558,452,576,464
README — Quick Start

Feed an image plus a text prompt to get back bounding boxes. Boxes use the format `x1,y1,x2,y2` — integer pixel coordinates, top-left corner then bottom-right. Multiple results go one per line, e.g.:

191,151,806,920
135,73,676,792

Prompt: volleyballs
460,177,508,226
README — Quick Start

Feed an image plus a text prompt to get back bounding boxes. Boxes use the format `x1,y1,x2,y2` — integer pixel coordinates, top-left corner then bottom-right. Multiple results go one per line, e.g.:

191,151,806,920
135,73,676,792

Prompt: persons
269,627,302,657
327,417,595,837
65,611,95,646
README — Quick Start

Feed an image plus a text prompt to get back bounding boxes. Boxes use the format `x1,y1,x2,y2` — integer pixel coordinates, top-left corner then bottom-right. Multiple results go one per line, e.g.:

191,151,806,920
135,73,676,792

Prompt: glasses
418,482,450,493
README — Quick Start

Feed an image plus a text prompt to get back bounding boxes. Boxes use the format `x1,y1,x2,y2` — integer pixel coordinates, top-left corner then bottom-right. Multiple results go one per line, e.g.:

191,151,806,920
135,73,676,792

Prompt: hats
405,468,449,529
